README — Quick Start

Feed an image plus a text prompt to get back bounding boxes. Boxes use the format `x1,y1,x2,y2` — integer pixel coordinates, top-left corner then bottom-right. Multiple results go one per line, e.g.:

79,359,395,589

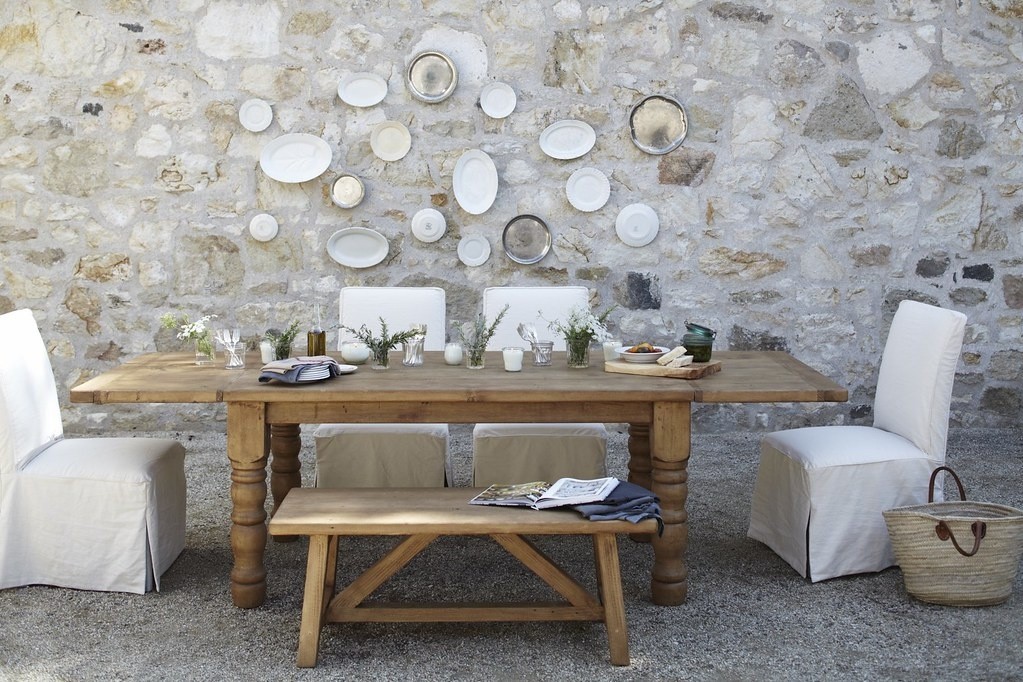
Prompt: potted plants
448,305,510,369
266,324,301,359
70,350,846,609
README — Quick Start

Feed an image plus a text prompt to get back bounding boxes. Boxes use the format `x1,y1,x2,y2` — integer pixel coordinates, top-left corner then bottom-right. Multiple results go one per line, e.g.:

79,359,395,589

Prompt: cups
223,342,246,369
530,340,554,366
603,342,621,361
502,347,524,371
259,341,271,363
444,343,462,364
341,339,371,364
402,339,424,365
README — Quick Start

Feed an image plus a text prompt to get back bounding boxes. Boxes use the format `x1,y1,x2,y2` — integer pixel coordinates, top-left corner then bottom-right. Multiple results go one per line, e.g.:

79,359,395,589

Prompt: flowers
535,295,614,349
161,310,217,342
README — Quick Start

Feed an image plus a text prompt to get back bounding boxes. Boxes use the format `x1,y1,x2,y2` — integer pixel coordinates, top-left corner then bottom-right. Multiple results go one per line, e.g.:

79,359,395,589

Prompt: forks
517,323,548,363
215,329,243,367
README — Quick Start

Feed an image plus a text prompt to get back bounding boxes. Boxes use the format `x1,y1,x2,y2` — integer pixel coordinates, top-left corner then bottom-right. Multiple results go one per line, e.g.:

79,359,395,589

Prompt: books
467,476,621,511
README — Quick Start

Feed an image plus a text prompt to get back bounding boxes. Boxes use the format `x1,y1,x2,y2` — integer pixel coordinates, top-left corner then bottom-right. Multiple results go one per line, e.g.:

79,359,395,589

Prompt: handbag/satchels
881,465,1023,606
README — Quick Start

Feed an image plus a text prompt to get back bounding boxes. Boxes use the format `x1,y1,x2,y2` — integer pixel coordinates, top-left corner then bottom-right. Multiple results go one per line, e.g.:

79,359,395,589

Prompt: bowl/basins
614,346,670,362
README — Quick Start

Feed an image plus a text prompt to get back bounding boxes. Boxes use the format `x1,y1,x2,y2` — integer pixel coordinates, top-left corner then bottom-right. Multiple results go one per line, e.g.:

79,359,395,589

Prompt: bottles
308,300,326,356
680,320,717,361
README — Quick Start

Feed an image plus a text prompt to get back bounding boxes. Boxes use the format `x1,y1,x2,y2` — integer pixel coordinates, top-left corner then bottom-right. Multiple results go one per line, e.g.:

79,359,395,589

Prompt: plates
627,93,688,155
406,50,457,102
337,73,388,107
238,98,272,132
296,363,357,384
480,81,517,118
249,120,660,268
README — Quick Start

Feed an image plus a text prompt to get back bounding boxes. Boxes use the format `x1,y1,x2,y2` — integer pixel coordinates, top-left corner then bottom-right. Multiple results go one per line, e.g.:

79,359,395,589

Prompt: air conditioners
330,316,423,369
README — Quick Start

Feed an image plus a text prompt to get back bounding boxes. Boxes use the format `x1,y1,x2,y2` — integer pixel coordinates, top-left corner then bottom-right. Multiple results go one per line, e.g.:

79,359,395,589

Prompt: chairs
313,287,454,489
746,300,968,580
468,286,607,487
0,307,186,593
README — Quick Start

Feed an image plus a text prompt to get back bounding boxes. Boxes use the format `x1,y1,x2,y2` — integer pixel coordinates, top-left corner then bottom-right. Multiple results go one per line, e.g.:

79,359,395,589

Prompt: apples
625,342,662,353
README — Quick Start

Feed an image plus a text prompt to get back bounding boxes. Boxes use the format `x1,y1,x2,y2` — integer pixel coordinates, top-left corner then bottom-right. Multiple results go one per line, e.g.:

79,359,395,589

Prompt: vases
565,338,589,367
195,342,216,367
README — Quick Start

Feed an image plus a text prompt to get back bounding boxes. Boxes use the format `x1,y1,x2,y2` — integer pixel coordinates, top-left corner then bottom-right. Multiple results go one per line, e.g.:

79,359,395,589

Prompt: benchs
270,489,660,666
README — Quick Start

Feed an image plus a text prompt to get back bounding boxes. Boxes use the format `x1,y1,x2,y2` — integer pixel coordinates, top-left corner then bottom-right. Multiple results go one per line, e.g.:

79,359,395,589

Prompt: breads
656,346,694,368
